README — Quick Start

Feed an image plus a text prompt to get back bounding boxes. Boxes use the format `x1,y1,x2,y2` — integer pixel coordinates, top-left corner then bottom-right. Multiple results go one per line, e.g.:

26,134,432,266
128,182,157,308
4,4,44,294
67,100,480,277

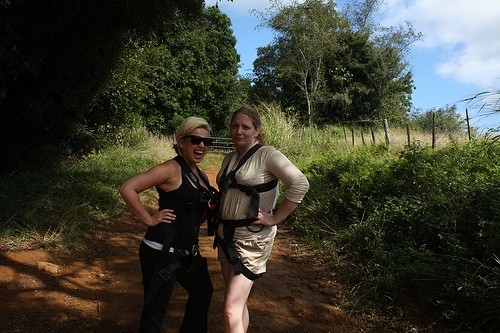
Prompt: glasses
181,135,213,147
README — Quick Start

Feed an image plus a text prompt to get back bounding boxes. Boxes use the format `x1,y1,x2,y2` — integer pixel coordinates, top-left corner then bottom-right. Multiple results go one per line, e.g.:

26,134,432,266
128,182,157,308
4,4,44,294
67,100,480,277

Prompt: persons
214,107,310,333
119,116,214,333
